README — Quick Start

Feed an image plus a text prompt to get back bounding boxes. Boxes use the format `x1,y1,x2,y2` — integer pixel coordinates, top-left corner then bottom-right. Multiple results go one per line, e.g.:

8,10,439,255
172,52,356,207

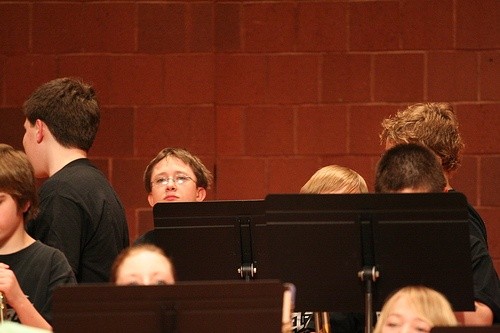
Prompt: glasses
150,175,199,185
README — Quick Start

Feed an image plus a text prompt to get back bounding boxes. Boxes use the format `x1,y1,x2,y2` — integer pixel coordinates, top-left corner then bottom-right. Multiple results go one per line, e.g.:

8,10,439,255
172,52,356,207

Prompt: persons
372,285,458,333
22,76,129,283
0,143,77,332
108,242,175,285
375,101,500,327
298,164,369,193
129,146,214,245
